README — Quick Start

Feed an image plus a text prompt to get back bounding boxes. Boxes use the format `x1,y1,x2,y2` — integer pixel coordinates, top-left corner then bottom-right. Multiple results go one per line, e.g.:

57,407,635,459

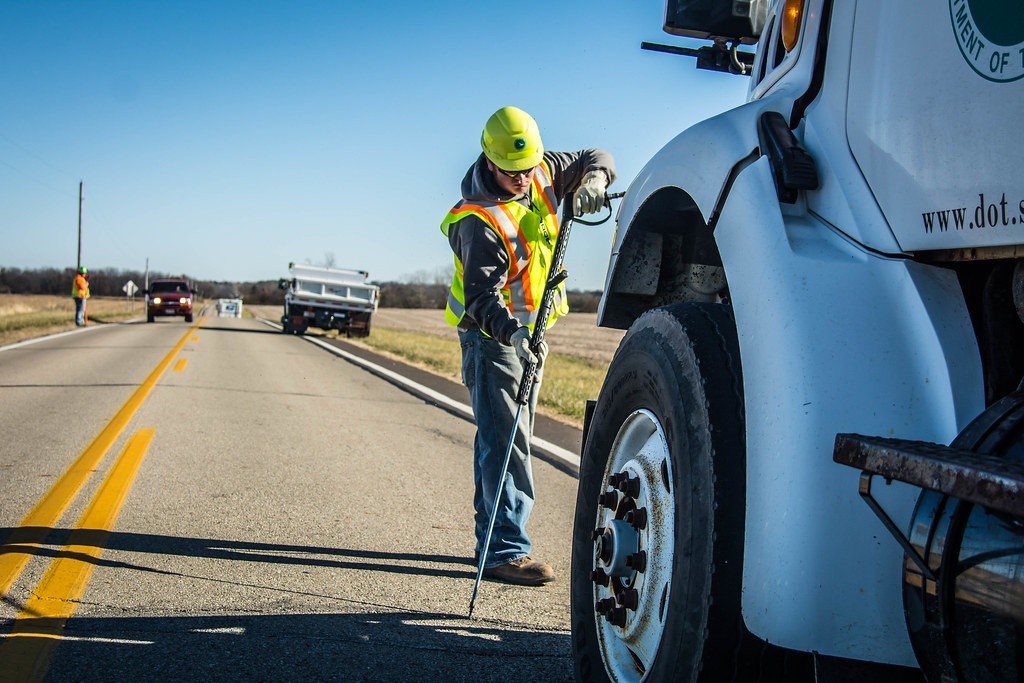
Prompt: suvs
142,280,197,323
569,0,1024,682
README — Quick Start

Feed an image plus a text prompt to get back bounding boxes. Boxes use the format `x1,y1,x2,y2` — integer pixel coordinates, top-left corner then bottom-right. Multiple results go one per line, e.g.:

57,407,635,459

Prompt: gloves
572,171,609,217
511,328,550,383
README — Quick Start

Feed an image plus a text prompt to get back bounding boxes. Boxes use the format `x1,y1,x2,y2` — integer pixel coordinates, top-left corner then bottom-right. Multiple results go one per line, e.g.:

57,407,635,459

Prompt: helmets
480,104,544,171
79,267,88,274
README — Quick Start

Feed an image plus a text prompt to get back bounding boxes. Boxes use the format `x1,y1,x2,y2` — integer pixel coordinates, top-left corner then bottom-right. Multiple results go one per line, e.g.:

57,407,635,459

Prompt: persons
71,266,90,326
440,105,616,587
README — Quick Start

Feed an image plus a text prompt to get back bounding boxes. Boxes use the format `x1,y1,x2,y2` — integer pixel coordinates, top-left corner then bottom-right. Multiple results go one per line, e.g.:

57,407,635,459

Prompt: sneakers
476,547,554,586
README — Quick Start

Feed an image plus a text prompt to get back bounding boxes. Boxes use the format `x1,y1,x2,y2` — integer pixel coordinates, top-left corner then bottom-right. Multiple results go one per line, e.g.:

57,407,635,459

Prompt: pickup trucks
276,263,384,338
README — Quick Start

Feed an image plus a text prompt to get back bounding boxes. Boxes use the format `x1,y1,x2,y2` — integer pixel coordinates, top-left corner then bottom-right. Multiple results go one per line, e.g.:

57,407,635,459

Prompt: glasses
497,165,533,180
